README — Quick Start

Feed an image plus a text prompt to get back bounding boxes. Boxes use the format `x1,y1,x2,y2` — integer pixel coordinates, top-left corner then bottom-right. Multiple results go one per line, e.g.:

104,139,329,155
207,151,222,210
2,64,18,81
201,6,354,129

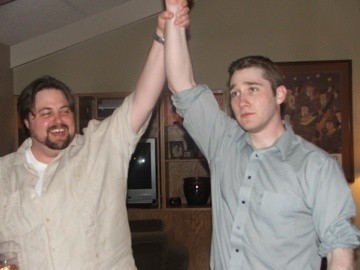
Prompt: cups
169,197,181,208
0,251,20,270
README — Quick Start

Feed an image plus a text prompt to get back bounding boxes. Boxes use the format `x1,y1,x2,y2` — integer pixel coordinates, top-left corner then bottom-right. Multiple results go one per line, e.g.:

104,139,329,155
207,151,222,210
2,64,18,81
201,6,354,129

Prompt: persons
164,0,360,270
1,1,191,270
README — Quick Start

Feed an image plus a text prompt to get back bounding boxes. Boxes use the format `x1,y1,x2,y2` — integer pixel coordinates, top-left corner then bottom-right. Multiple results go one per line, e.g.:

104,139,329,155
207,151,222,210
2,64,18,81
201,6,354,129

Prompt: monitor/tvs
126,138,157,207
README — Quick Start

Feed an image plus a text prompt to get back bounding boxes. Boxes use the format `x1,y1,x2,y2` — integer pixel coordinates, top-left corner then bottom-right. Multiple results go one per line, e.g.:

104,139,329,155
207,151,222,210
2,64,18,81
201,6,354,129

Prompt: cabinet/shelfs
13,89,229,270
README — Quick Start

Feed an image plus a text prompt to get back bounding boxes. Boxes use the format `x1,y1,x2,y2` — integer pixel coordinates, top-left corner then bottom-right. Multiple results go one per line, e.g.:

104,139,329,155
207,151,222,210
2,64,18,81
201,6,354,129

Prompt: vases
183,177,212,207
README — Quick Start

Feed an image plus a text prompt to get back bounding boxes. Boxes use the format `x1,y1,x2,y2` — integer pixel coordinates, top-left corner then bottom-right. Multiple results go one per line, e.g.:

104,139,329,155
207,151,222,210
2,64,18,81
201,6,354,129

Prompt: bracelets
153,31,165,44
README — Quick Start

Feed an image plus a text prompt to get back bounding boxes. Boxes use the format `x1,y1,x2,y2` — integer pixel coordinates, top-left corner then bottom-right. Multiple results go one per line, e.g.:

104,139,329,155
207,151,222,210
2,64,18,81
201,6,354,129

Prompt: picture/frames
170,141,184,159
275,59,354,183
